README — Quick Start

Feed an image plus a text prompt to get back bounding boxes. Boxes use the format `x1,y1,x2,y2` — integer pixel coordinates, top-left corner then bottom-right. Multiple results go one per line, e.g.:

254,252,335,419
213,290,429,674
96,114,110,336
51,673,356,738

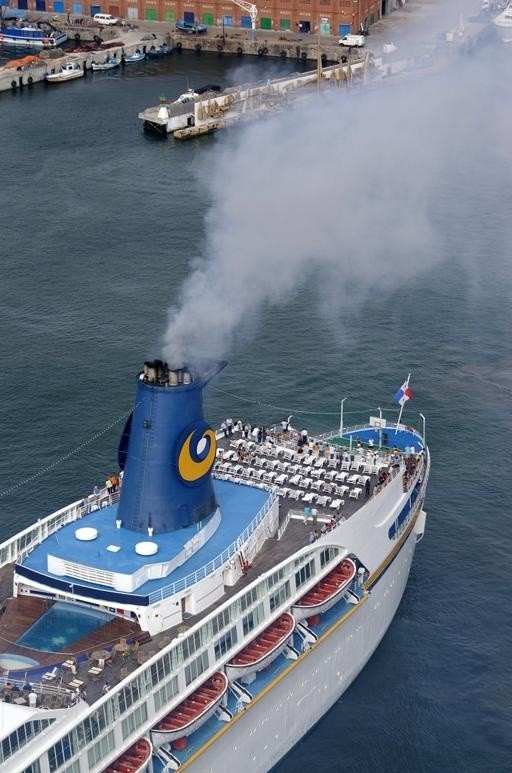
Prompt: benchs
227,615,293,666
105,739,149,773
296,564,351,607
152,674,230,732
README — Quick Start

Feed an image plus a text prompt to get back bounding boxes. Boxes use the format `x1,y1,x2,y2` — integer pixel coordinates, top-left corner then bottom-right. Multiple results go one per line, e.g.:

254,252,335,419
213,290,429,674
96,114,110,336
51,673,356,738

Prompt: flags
393,381,413,407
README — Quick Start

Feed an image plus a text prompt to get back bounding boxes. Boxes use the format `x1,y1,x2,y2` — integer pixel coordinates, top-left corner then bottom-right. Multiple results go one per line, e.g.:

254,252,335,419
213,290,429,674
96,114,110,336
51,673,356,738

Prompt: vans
339,33,365,47
93,12,117,25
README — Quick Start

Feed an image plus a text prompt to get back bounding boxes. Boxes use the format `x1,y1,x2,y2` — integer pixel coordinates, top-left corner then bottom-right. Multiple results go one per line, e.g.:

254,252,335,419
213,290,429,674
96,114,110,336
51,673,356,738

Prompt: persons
302,505,343,542
102,682,109,694
357,563,366,587
217,417,269,464
15,15,22,28
355,435,418,495
0,681,88,708
280,418,331,460
81,470,124,507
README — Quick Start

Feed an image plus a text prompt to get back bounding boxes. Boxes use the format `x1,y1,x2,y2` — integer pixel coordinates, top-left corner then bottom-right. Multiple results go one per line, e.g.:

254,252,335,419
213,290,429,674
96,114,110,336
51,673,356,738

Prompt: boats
91,57,121,72
148,42,174,56
0,5,68,48
47,60,84,84
0,355,433,773
171,81,222,103
123,51,146,63
493,0,511,43
174,20,207,34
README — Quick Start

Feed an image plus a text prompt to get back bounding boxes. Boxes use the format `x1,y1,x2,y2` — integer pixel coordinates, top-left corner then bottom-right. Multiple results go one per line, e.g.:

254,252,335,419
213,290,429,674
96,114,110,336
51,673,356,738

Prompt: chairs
210,437,384,511
4,635,171,708
